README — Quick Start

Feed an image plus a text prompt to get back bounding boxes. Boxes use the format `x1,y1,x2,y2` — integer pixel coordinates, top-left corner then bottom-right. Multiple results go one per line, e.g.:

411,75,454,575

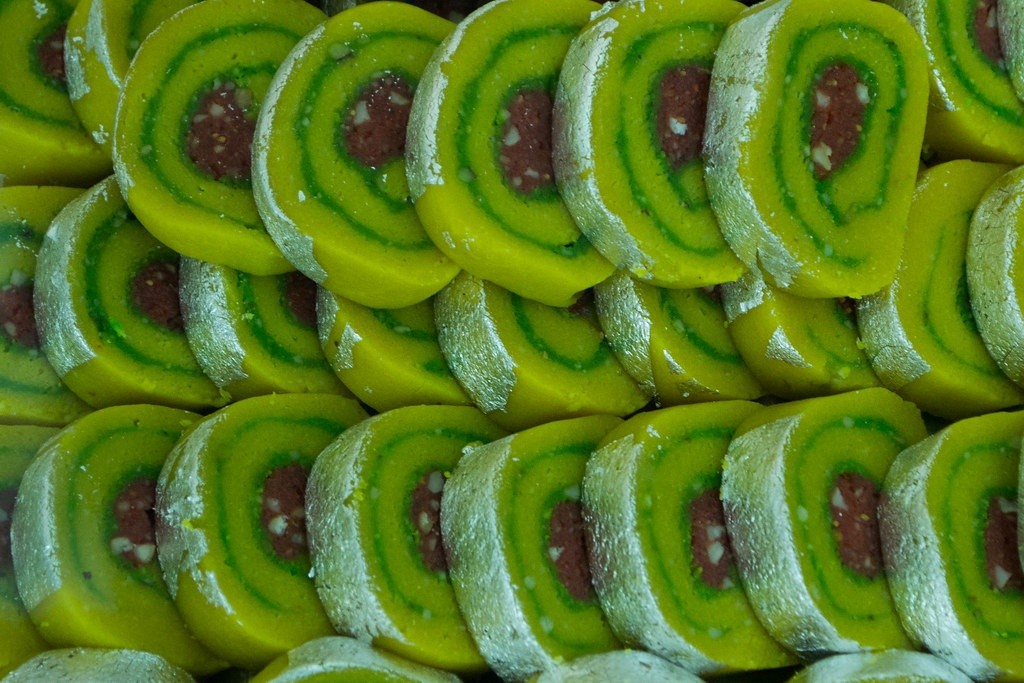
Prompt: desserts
0,0,1024,683
